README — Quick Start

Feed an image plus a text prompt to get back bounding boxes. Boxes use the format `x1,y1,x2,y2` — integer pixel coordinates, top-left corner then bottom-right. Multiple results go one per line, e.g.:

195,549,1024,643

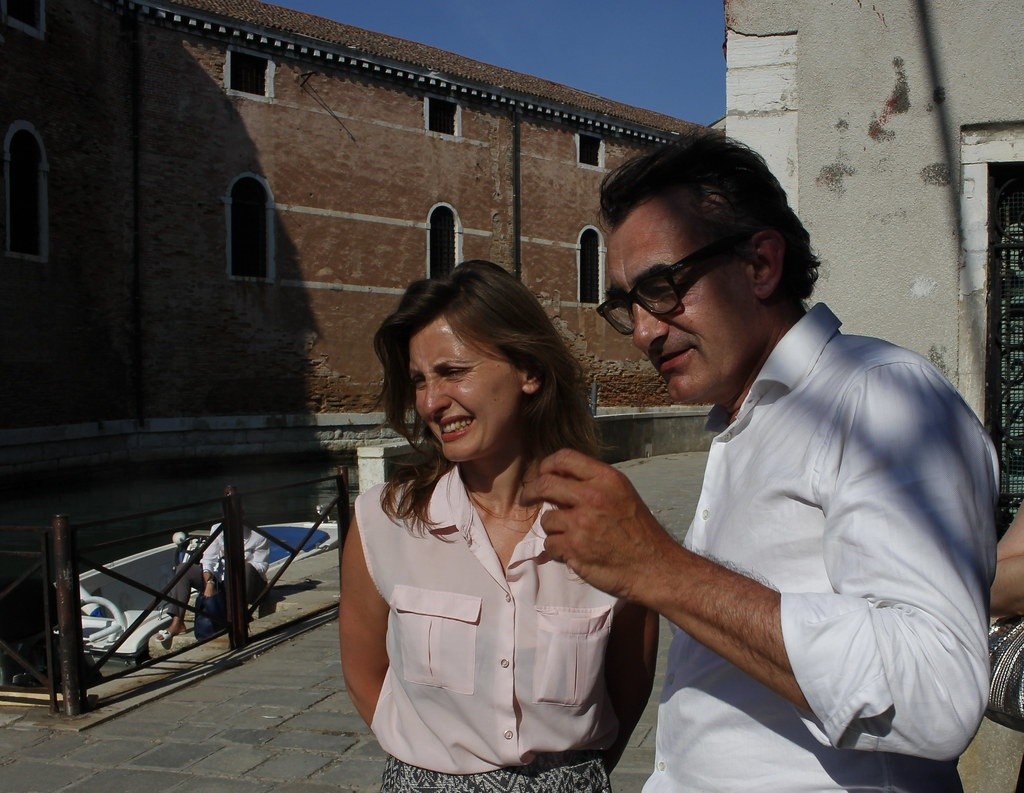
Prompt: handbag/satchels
194,557,227,640
984,615,1024,732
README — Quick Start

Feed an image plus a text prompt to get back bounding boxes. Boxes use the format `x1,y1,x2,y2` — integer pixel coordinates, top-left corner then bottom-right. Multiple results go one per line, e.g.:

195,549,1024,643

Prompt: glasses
596,226,767,335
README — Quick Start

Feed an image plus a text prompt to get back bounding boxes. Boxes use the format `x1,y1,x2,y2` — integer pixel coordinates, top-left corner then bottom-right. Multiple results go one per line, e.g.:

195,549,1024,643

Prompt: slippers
156,630,186,642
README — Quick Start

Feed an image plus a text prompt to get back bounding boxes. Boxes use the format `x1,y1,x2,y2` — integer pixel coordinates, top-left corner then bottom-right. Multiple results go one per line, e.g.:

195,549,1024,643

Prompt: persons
989,497,1024,793
518,131,999,793
153,501,271,642
333,260,661,793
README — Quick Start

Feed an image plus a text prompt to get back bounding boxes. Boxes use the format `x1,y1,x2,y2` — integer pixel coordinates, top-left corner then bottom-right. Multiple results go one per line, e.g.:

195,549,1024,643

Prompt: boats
53,506,340,676
